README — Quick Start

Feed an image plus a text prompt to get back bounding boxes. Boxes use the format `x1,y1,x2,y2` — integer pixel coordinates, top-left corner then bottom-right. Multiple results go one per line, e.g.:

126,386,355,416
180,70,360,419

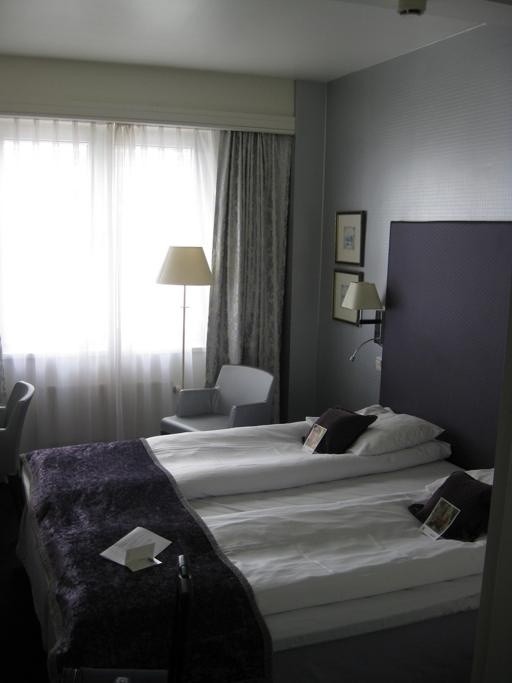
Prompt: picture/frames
333,210,366,267
331,268,364,328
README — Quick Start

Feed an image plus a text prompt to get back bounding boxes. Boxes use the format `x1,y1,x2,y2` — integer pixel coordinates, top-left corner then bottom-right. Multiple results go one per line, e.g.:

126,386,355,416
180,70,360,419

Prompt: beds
19,414,495,681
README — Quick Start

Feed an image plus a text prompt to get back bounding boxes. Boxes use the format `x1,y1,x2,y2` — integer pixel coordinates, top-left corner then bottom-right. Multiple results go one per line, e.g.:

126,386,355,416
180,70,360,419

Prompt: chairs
0,379,35,486
159,363,277,435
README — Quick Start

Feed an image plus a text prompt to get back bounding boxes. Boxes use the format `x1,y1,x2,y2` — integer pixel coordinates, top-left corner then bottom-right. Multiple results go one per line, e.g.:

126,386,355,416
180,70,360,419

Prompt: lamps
168,246,212,389
341,280,384,363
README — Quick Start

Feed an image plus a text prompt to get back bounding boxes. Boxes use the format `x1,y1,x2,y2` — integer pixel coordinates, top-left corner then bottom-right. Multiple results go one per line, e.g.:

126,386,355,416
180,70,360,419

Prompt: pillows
300,404,446,458
408,467,494,542
